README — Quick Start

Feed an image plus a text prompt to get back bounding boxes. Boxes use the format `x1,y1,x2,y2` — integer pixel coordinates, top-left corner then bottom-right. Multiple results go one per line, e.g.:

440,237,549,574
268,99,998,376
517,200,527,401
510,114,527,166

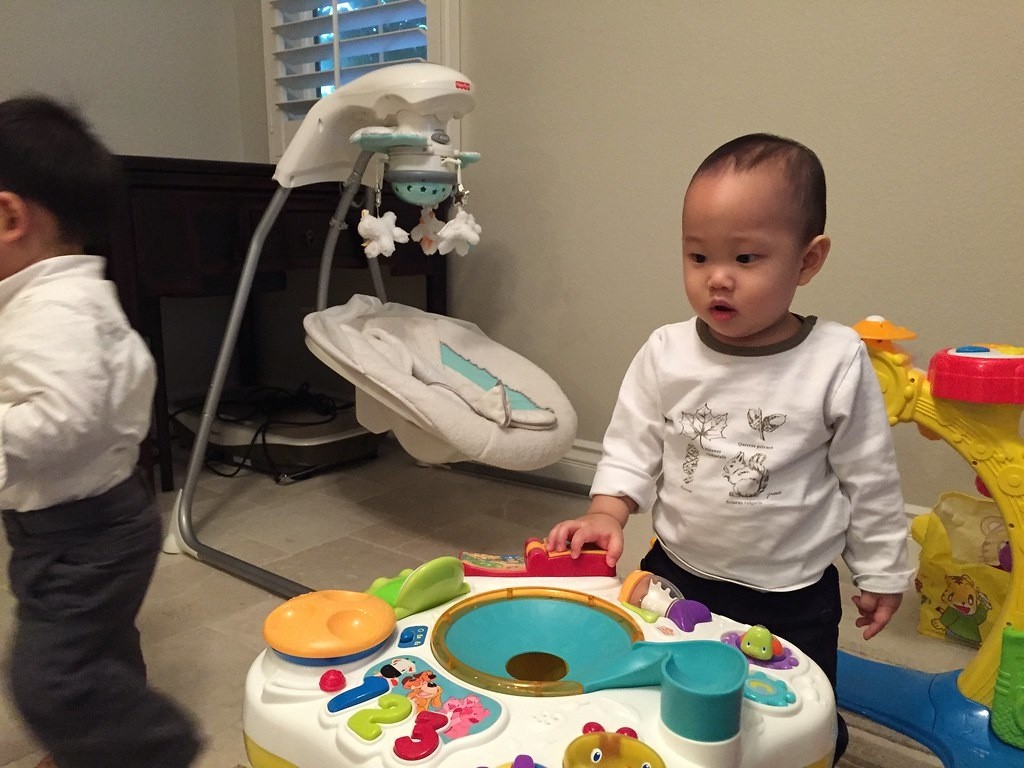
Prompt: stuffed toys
357,206,482,258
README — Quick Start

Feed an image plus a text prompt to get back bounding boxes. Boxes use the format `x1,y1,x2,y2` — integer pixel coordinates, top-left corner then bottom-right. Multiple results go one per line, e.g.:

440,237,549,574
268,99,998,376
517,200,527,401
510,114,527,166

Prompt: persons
0,97,212,768
546,135,920,768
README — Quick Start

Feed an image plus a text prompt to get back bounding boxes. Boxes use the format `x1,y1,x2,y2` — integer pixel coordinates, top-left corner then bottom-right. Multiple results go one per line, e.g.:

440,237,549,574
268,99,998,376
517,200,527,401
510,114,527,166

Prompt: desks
80,152,448,493
